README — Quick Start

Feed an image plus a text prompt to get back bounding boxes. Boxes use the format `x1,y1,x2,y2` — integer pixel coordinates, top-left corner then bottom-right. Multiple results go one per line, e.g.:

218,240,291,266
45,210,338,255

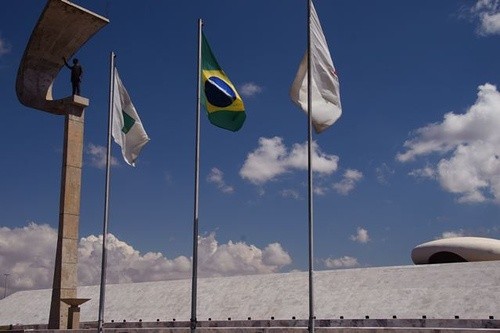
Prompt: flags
290,0,343,133
111,66,150,167
201,29,247,131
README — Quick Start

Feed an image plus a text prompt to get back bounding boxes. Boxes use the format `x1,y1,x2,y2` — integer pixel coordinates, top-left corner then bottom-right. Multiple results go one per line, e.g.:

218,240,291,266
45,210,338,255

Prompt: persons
62,55,84,95
